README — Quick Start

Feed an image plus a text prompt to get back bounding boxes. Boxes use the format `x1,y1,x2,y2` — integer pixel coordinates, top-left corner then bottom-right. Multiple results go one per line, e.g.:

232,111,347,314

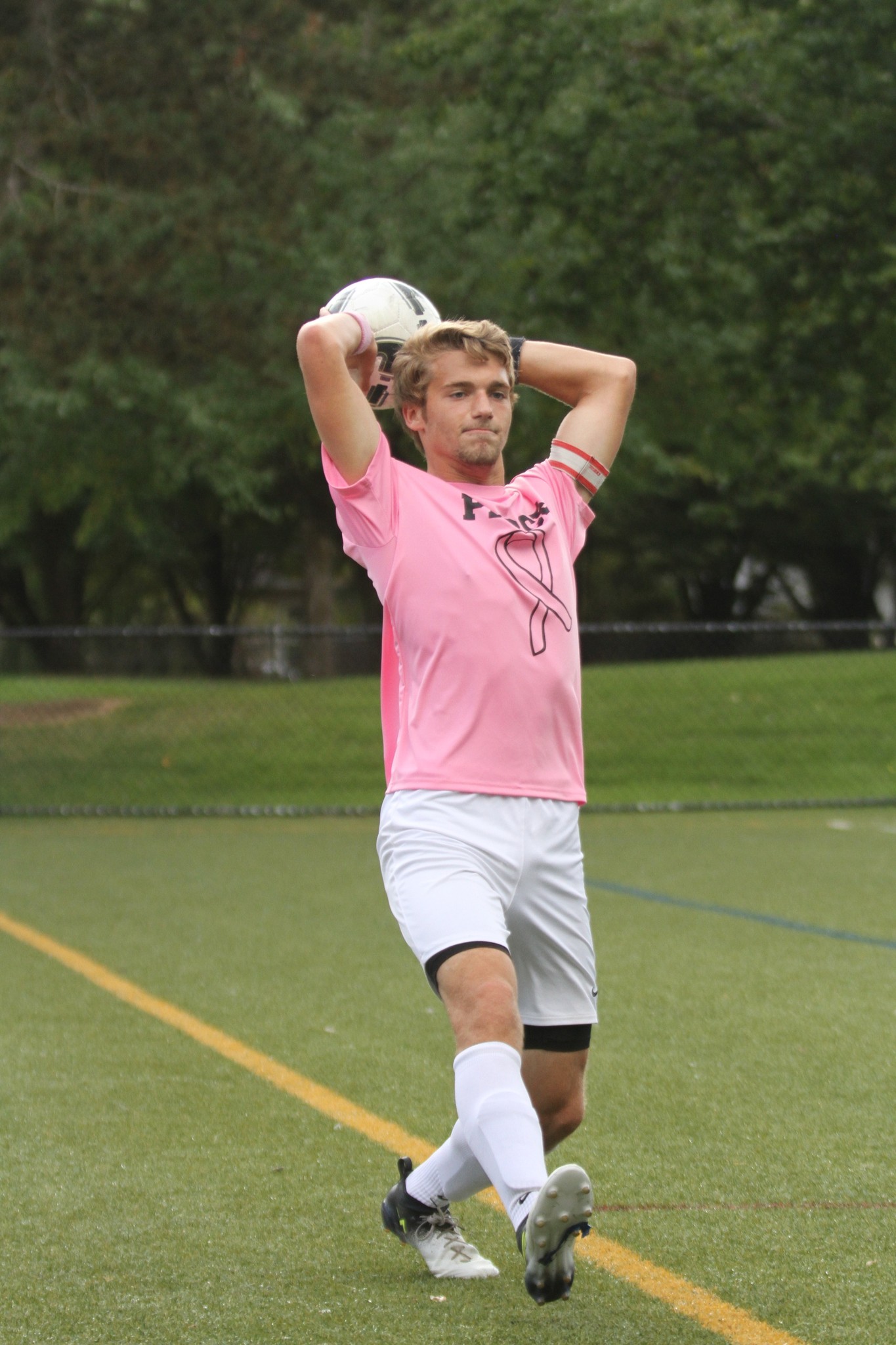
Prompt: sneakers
380,1159,499,1280
513,1163,595,1305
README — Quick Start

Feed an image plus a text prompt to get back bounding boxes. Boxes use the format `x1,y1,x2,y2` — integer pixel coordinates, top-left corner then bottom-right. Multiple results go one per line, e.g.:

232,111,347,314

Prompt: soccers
319,275,443,410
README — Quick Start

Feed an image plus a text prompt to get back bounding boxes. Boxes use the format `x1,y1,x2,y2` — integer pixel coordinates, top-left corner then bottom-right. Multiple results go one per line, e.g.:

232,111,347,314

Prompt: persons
297,311,636,1310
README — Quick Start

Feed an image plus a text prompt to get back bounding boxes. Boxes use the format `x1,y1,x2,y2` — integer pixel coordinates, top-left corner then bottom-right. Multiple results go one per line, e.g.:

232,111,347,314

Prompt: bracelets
508,337,526,384
344,310,373,356
